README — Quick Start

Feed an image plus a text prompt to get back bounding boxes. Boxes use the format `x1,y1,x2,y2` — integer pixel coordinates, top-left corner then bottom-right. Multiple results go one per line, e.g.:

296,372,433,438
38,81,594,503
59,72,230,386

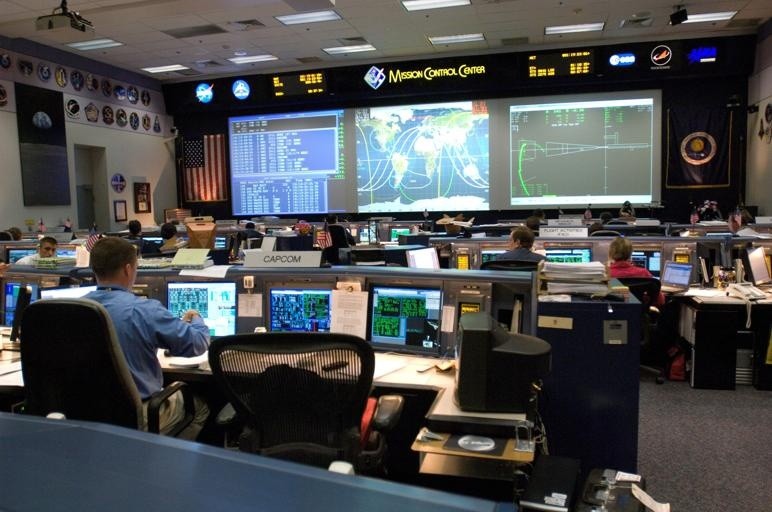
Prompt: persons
229,222,264,251
496,225,548,301
294,216,355,266
687,199,753,238
76,237,210,442
584,201,664,307
120,220,179,257
13,236,56,267
1,227,22,266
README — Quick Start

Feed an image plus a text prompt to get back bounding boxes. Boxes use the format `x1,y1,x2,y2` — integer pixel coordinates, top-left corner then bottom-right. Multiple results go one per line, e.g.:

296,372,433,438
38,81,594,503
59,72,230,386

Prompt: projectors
35,14,96,43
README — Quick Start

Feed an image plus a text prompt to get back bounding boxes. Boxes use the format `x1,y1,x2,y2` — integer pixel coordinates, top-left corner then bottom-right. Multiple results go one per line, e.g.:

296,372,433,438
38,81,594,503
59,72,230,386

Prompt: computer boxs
735,329,756,386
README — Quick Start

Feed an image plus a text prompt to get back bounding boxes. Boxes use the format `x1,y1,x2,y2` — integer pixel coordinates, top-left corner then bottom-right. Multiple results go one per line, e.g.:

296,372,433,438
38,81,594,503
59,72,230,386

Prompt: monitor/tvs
405,247,441,270
213,235,227,249
165,281,238,337
388,225,411,240
482,250,508,262
4,281,40,325
631,250,662,276
142,236,164,248
6,248,38,264
749,246,770,285
545,248,592,264
270,288,332,332
359,226,375,242
55,248,77,259
366,282,443,359
456,313,551,412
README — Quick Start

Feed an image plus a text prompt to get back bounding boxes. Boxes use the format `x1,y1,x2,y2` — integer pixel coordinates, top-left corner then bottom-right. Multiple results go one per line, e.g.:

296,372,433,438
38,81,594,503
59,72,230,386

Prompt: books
135,259,169,268
171,248,213,269
38,257,75,270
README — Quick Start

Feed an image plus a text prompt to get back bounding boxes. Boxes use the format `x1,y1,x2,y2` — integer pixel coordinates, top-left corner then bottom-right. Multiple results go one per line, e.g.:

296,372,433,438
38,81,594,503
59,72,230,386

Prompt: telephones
725,281,768,300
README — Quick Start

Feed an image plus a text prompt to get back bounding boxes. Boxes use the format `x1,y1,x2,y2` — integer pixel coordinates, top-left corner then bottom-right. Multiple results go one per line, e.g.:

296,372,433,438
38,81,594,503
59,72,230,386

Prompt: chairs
208,331,406,482
1,222,770,388
20,298,196,441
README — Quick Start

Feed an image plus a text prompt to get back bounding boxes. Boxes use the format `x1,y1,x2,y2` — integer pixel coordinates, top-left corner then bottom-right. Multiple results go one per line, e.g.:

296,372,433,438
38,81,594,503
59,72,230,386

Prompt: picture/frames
114,199,129,222
133,181,153,214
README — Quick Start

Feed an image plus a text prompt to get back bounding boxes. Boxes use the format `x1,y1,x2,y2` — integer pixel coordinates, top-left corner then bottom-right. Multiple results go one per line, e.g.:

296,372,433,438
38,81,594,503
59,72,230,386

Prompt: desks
0,346,534,503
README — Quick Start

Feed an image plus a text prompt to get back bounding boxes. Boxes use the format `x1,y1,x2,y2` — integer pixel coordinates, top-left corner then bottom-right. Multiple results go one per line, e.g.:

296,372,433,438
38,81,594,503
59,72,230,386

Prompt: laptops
660,260,693,293
